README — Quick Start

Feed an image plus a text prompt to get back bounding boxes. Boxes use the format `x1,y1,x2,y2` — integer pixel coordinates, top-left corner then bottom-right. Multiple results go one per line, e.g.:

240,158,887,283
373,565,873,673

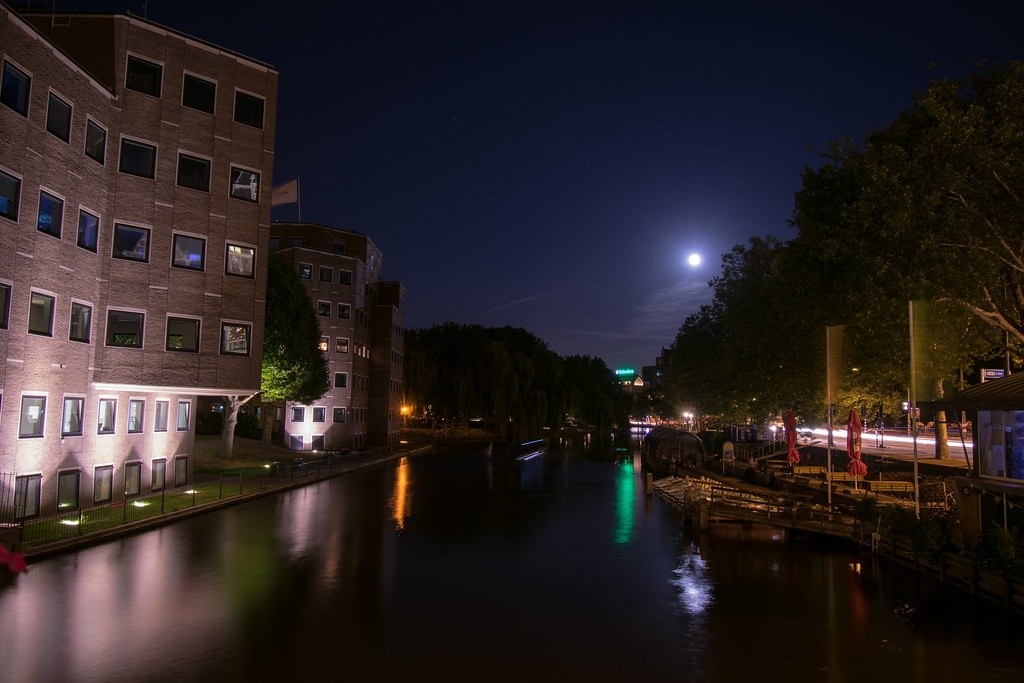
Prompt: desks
765,460,795,473
822,472,864,488
792,466,827,478
864,480,914,501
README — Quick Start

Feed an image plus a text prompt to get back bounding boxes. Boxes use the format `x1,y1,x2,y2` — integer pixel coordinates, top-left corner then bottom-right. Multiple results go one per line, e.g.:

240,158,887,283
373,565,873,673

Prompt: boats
640,426,709,481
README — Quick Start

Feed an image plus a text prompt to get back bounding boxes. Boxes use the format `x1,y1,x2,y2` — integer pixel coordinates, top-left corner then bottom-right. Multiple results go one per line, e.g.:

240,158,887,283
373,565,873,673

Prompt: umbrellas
784,411,799,467
847,409,866,489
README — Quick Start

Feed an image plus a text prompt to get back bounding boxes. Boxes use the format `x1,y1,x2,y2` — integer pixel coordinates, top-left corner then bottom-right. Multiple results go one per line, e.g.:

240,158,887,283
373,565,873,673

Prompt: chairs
919,482,956,509
